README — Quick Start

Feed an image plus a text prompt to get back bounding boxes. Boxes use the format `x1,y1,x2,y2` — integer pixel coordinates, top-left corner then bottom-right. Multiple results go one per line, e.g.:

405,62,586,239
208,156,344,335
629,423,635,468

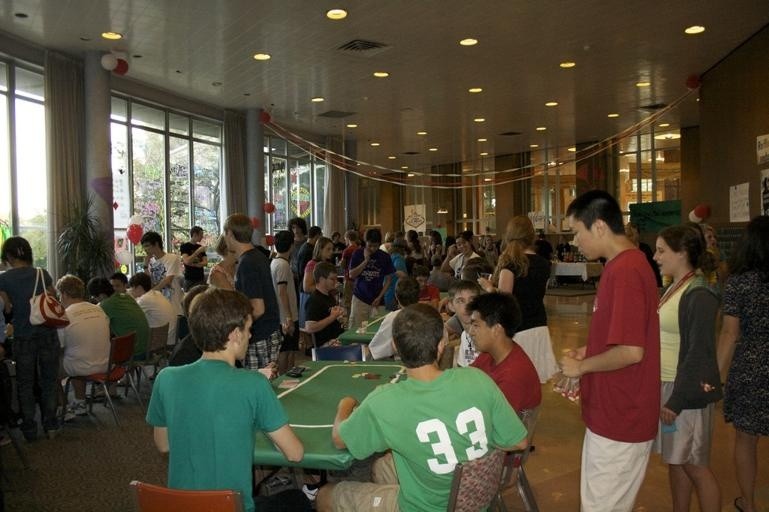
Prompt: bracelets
721,383,726,387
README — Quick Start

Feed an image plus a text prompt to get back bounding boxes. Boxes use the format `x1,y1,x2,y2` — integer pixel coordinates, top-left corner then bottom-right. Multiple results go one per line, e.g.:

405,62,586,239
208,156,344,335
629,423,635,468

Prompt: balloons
113,59,129,76
263,203,276,214
694,204,711,218
686,74,700,89
100,53,119,71
259,112,271,124
688,211,703,224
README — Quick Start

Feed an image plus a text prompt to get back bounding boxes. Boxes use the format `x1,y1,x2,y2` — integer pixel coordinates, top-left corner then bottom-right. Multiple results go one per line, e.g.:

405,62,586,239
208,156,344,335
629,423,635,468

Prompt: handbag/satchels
29,291,72,327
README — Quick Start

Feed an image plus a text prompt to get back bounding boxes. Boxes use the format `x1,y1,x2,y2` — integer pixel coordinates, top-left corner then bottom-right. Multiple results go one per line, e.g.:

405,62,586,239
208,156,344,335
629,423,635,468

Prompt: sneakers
301,480,332,502
0,390,122,449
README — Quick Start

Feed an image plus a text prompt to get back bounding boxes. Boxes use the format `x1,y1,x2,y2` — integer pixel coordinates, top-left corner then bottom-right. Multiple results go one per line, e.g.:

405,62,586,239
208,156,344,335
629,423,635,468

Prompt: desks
552,262,604,290
337,292,454,361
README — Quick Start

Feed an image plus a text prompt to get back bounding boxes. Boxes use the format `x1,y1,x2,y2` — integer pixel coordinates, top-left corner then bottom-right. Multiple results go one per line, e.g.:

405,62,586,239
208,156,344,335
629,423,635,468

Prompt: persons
0,212,572,512
622,223,664,287
652,222,724,511
700,224,728,291
558,187,661,512
714,214,768,511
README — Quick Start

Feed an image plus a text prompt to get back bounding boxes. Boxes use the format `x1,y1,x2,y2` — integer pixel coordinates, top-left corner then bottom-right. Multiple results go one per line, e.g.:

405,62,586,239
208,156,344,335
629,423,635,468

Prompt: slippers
733,495,758,512
